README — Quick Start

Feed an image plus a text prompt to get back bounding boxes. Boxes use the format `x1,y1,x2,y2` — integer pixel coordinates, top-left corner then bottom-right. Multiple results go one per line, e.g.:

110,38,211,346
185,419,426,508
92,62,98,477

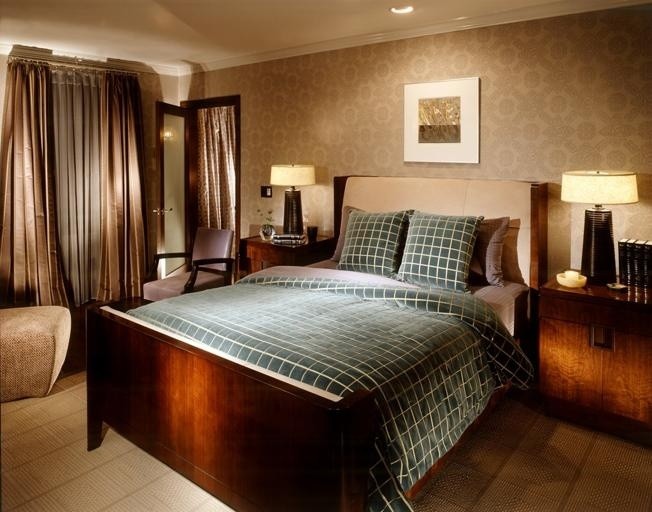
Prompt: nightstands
241,233,332,275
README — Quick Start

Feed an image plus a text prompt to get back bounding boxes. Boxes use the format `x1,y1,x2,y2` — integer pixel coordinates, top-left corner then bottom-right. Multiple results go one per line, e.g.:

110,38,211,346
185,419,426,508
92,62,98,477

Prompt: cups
307,225,317,241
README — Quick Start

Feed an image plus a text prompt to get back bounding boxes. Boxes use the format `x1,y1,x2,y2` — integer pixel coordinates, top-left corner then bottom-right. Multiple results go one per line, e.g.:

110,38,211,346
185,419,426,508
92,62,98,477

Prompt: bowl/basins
556,273,587,288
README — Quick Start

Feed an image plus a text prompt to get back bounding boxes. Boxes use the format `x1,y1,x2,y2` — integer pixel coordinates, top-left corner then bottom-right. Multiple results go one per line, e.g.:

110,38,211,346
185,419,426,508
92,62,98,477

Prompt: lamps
560,169,639,285
270,163,315,235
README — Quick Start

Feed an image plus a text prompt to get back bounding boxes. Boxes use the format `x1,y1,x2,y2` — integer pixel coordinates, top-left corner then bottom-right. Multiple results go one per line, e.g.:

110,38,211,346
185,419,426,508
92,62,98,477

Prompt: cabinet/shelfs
539,267,652,446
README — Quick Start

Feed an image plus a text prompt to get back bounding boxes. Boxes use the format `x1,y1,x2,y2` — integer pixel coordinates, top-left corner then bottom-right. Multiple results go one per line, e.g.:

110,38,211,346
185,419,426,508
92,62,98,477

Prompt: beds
86,175,547,512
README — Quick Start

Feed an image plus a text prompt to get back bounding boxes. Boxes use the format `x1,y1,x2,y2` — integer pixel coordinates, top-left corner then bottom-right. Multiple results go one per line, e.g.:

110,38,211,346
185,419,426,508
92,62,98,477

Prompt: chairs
143,227,235,305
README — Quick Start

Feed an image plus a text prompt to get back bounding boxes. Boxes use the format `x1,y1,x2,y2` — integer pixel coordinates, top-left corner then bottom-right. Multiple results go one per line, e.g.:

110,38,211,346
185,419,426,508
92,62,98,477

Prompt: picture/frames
402,77,480,165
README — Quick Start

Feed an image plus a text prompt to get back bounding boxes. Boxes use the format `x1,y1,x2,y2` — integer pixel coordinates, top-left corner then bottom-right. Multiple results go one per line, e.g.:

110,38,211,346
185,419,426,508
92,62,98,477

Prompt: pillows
331,205,510,293
